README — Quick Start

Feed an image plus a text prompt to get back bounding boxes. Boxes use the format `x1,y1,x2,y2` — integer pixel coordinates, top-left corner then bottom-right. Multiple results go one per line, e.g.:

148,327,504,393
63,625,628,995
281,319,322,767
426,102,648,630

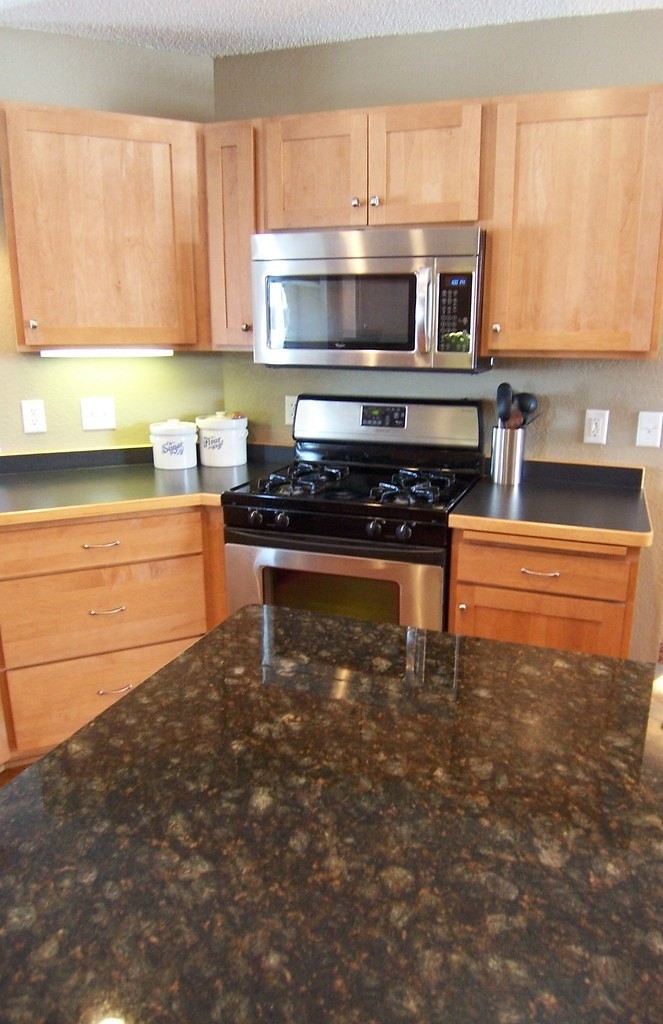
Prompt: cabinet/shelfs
0,442,653,775
0,87,663,359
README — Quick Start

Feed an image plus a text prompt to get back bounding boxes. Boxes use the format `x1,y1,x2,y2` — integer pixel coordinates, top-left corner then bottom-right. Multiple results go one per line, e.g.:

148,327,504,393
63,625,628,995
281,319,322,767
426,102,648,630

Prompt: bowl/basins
197,410,249,467
149,419,197,470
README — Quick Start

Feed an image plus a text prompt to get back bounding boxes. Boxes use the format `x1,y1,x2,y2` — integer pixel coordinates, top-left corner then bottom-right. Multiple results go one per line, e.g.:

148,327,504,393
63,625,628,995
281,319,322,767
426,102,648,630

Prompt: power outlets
20,399,46,434
583,409,609,444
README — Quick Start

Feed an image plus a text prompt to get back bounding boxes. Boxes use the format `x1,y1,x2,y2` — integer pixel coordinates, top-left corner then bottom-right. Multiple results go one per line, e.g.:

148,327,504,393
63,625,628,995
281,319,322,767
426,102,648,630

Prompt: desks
0,602,663,1024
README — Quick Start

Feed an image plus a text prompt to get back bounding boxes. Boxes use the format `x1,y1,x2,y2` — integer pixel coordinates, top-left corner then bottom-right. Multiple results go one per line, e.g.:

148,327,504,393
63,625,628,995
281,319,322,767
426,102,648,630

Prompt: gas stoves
222,395,485,565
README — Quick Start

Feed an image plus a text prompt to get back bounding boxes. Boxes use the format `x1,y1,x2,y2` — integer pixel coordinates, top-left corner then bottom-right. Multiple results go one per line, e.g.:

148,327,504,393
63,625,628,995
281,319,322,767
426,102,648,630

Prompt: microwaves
250,226,495,374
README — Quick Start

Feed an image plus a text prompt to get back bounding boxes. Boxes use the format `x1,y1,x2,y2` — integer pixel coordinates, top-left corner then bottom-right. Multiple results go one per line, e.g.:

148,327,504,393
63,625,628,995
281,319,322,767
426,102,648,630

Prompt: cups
490,427,524,485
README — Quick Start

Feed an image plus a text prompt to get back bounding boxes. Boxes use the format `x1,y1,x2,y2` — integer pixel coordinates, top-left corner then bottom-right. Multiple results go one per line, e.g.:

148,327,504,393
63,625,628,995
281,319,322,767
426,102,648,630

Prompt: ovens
223,543,443,634
